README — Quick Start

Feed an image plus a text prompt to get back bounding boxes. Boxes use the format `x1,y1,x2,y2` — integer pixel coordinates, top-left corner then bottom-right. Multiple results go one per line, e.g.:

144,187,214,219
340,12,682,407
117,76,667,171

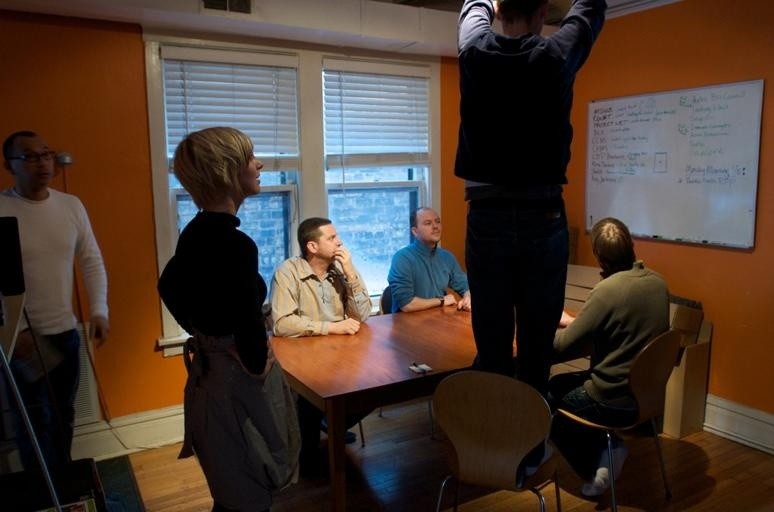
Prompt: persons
449,0,607,474
386,205,470,316
546,214,672,500
263,217,374,463
0,130,111,474
156,125,304,512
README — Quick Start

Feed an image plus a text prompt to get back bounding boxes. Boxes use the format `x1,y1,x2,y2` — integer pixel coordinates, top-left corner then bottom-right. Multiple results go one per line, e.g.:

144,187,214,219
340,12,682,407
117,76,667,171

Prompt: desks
269,304,567,512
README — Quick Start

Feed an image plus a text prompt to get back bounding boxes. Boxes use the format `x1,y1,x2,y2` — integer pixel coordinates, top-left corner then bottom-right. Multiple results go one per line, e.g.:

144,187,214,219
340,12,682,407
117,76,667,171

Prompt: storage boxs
663,320,726,439
669,294,703,347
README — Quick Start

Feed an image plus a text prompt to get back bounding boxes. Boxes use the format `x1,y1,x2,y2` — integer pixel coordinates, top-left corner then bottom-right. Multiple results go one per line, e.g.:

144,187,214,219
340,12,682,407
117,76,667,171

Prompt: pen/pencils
413,363,426,372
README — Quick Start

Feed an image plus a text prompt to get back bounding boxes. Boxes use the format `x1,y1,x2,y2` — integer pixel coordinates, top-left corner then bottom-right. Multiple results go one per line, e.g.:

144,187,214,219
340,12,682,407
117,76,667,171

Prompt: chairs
555,329,688,512
378,286,392,417
263,303,365,447
429,371,552,512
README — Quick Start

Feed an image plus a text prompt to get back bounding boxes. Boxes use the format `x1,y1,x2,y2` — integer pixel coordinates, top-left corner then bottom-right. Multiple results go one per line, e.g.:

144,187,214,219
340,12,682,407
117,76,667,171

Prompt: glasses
4,151,54,162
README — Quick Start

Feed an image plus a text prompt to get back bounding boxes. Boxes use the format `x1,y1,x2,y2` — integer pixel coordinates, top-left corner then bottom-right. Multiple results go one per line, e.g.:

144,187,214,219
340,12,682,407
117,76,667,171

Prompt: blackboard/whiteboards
585,79,765,250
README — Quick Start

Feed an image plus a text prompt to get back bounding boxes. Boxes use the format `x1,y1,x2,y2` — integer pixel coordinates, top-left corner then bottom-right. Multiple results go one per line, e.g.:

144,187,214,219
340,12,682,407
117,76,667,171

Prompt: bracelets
437,294,445,307
